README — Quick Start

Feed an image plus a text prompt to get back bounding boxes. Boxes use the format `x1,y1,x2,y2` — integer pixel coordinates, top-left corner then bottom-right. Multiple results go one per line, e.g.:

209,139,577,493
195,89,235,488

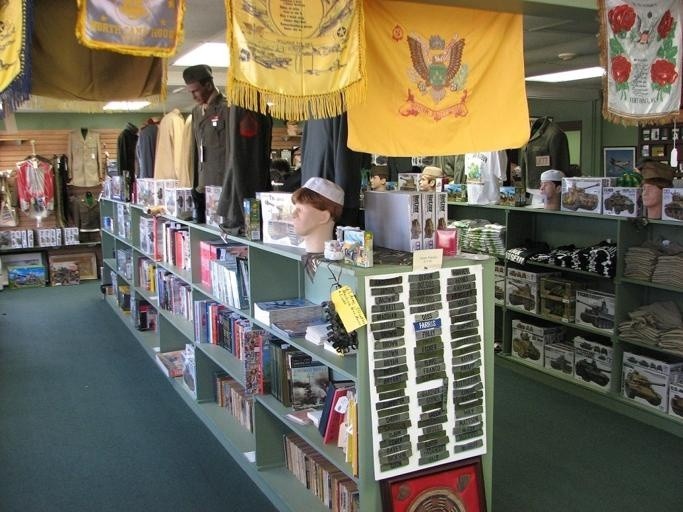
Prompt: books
199,236,250,308
242,329,358,476
216,373,253,431
254,298,357,356
158,268,193,322
282,431,358,512
161,220,190,271
195,294,252,361
137,255,156,296
155,348,187,377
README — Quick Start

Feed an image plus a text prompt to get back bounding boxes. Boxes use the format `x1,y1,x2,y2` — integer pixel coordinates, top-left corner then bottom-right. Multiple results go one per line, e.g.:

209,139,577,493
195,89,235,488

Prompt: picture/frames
48,252,98,281
1,250,50,286
603,147,636,177
379,456,486,512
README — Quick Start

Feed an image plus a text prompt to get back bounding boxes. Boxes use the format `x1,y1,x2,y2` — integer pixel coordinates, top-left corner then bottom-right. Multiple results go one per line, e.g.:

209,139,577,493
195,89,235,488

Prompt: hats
182,65,214,85
540,169,566,182
301,177,345,207
641,160,675,180
370,166,390,177
270,158,290,173
421,166,443,177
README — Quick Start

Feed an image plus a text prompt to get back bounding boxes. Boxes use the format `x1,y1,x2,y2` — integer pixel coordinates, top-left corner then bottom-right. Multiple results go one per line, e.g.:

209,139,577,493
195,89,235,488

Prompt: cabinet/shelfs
447,200,506,354
502,207,620,393
99,198,496,512
611,218,683,427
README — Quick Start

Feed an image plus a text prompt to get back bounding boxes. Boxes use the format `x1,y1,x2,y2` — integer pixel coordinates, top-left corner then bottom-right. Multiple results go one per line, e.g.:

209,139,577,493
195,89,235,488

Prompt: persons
291,176,345,255
539,170,565,209
419,167,442,192
371,166,388,191
641,160,674,220
183,64,228,222
